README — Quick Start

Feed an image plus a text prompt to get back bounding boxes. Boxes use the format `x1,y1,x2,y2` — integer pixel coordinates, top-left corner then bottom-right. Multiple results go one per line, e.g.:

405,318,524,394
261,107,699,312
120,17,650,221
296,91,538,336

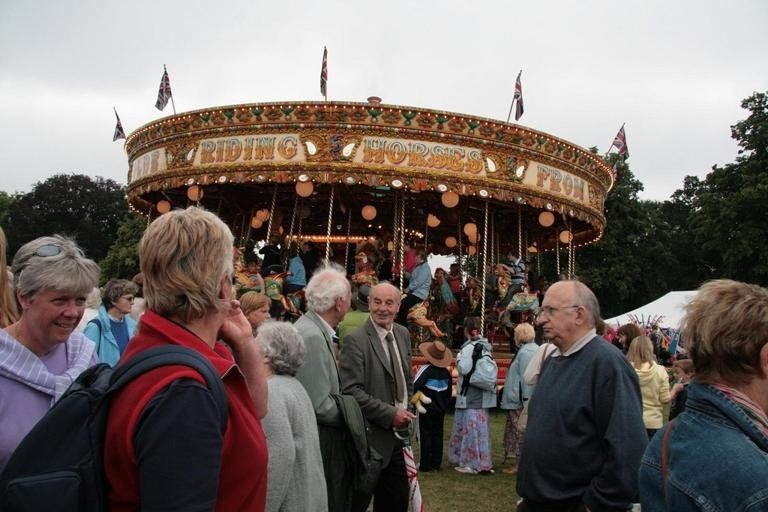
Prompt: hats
418,340,453,368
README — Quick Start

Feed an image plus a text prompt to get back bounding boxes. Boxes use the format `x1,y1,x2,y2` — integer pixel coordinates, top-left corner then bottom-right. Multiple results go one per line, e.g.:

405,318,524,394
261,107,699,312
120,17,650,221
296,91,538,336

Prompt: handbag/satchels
469,343,499,391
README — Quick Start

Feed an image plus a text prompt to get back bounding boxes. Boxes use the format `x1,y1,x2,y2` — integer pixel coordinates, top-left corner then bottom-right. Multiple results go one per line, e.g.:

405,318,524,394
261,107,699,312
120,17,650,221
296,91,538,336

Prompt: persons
389,240,768,512
2,207,425,511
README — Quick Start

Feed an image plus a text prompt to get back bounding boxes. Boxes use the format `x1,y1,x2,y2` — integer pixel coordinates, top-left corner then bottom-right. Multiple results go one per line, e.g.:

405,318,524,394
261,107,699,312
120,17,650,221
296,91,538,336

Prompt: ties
386,332,404,403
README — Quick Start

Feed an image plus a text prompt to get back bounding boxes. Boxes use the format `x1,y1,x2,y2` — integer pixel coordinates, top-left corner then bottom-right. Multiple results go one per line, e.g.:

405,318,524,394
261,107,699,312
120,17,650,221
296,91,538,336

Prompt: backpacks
0,345,229,512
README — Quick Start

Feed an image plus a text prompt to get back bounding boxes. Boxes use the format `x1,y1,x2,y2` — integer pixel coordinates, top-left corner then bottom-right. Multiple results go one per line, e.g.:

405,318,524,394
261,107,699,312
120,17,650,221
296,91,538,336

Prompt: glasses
30,244,61,257
535,306,578,317
120,297,134,301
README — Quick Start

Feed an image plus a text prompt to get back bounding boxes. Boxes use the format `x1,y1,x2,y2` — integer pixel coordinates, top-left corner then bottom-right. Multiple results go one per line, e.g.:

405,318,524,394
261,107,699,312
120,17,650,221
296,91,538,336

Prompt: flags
614,127,629,161
113,110,125,141
514,76,523,120
320,49,327,96
155,71,172,111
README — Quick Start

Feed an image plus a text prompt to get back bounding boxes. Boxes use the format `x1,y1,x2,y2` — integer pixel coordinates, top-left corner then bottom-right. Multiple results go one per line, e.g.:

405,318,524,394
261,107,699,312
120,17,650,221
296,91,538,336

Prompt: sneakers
454,466,518,475
